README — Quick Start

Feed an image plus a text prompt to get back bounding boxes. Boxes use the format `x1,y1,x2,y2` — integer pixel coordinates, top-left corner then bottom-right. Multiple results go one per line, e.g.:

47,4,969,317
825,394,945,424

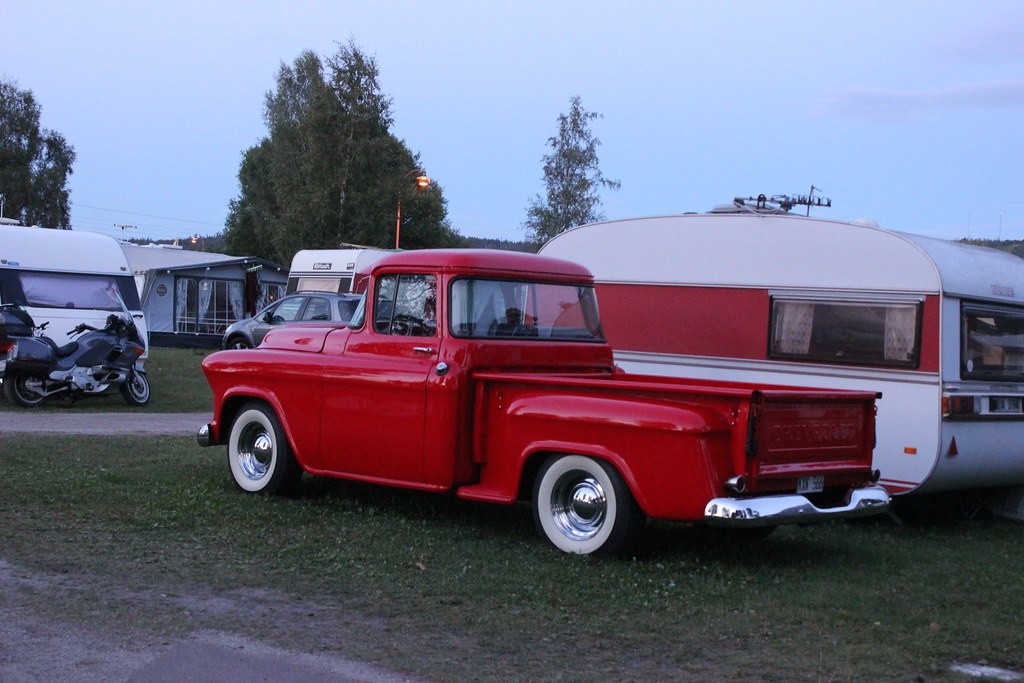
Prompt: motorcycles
0,288,152,409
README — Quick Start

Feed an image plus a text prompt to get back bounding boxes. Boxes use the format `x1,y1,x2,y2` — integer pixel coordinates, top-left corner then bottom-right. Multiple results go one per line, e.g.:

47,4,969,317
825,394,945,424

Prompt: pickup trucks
198,248,893,560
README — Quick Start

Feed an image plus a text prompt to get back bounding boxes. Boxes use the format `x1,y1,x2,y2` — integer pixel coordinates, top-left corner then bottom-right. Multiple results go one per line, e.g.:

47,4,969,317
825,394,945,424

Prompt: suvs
221,290,393,351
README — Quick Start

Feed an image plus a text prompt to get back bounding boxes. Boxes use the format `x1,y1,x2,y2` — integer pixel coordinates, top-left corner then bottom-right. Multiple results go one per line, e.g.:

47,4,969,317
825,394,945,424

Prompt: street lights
395,169,431,249
191,234,204,252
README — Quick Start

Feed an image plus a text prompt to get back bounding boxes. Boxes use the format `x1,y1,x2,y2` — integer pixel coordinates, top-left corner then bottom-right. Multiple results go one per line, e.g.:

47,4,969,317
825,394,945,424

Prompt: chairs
314,302,326,313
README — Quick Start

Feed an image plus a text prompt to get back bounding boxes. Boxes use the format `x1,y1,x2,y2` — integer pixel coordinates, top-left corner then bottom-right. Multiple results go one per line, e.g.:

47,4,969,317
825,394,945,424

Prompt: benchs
457,323,593,337
